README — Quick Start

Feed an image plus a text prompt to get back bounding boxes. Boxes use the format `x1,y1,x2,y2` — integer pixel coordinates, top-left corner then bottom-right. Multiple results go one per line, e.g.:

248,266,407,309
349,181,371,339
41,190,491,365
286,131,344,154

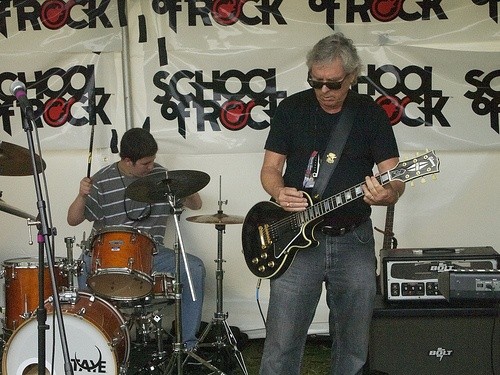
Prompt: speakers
365,294,500,375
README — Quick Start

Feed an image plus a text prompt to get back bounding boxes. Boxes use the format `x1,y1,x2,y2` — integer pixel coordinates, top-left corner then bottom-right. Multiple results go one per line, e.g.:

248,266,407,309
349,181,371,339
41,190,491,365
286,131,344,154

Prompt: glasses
306,70,351,89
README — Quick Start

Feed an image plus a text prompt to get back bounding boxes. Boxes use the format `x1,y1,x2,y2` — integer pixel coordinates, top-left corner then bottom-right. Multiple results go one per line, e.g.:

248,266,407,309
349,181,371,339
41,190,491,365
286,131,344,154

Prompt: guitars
240,148,442,280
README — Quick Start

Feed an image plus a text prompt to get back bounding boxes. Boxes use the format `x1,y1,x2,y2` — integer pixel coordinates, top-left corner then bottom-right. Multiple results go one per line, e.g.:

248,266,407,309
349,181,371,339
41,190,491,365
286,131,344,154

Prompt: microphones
9,80,35,120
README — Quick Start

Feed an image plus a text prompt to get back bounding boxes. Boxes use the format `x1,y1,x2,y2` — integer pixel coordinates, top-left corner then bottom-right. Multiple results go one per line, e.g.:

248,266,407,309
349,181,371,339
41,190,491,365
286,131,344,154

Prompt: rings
369,196,373,200
286,202,290,207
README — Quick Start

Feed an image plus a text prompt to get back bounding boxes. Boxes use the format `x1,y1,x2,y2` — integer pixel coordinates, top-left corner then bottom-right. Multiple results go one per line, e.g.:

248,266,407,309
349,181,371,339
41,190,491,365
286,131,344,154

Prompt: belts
315,216,370,238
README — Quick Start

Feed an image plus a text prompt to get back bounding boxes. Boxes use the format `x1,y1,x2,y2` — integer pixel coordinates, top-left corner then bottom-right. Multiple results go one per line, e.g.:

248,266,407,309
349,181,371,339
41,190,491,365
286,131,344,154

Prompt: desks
368,294,500,375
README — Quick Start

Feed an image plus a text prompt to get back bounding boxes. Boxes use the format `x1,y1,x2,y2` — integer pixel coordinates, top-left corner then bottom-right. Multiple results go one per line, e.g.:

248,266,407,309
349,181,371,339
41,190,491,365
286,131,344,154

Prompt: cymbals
0,140,47,177
184,213,246,225
124,170,211,204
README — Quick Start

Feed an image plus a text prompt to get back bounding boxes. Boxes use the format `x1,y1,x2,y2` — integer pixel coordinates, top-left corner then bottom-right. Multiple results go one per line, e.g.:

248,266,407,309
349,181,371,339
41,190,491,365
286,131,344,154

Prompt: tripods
127,181,248,375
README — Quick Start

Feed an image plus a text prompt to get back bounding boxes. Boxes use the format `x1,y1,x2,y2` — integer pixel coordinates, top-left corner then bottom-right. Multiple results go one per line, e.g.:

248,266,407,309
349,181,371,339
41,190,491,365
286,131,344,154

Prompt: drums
153,271,180,303
86,225,158,304
0,291,131,375
2,256,74,340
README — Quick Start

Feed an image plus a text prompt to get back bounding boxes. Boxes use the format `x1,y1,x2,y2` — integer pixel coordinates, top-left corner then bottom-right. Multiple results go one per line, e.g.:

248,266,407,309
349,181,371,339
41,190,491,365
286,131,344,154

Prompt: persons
67,127,217,364
255,33,405,375
